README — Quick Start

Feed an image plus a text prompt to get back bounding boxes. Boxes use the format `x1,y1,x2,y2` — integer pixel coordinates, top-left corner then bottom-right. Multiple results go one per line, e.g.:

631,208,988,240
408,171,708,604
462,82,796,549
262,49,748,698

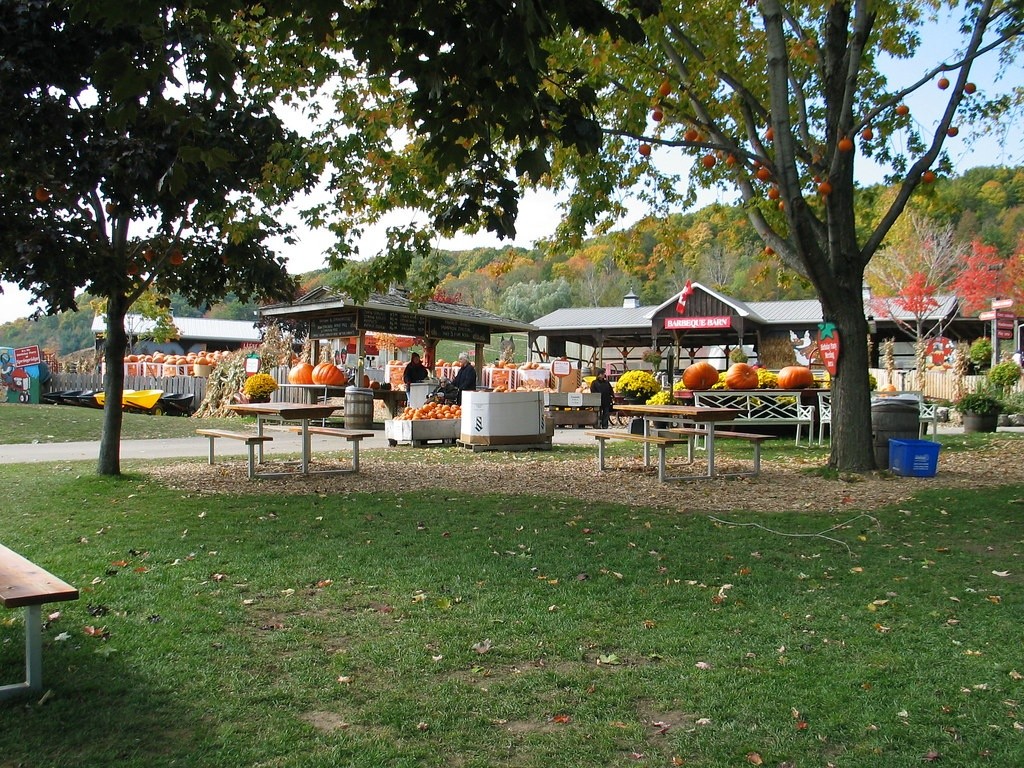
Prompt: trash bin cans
872,404,921,472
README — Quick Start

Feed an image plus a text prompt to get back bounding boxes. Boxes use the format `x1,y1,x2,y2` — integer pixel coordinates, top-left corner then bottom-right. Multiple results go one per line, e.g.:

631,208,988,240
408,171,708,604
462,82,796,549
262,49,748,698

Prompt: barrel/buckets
872,398,921,469
344,387,373,430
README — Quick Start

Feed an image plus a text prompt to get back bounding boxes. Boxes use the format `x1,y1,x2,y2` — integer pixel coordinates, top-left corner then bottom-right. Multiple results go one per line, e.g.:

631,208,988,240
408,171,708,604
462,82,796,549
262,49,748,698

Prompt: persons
403,352,428,407
590,368,614,429
428,352,476,406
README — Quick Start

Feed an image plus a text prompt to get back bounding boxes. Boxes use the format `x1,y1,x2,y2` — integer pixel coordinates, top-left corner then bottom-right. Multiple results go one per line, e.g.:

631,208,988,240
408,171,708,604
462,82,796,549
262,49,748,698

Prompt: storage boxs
384,363,551,391
384,419,462,441
887,437,942,478
566,391,601,407
460,389,546,436
99,362,194,378
544,393,569,407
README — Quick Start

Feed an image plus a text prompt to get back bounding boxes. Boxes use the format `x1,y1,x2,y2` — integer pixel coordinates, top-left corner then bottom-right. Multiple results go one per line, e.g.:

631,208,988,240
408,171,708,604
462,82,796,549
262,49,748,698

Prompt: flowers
645,388,684,406
642,349,663,364
760,384,777,410
672,367,879,410
612,369,662,394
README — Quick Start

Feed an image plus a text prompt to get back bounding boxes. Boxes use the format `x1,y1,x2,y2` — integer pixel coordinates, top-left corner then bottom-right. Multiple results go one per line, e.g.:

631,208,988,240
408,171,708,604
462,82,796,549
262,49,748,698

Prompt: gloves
428,393,434,399
436,392,444,398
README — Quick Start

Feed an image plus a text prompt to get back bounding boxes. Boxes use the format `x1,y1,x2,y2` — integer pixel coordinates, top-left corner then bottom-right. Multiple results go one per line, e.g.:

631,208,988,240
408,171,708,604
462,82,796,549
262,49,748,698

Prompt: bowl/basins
193,364,212,376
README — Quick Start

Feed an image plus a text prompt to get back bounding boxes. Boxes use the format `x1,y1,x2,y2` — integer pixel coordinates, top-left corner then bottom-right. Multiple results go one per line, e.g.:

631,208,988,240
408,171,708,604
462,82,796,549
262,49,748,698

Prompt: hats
558,351,567,357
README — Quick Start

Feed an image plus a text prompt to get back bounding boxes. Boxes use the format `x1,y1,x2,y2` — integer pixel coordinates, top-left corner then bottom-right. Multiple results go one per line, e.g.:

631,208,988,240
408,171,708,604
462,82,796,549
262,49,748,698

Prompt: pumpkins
492,385,517,392
777,365,813,390
288,362,344,386
392,401,462,419
363,375,392,390
879,384,896,396
387,359,463,367
725,362,758,388
497,360,545,369
124,350,224,374
683,361,719,390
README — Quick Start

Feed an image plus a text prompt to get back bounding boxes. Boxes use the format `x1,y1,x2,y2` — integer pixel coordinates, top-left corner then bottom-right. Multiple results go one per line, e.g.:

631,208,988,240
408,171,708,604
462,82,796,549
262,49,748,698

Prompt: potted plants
936,400,1024,427
728,347,749,363
955,381,1006,433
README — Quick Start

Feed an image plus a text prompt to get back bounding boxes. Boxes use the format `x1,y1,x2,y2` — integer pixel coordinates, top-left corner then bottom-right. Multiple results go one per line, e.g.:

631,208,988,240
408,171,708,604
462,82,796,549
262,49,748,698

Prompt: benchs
288,425,374,473
669,428,778,480
0,543,80,695
692,392,816,450
816,390,938,449
585,427,688,482
196,427,274,478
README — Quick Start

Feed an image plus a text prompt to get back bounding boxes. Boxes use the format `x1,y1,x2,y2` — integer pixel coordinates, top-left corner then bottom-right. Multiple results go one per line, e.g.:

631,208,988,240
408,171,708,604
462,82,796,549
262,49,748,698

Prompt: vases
623,389,655,413
650,412,670,429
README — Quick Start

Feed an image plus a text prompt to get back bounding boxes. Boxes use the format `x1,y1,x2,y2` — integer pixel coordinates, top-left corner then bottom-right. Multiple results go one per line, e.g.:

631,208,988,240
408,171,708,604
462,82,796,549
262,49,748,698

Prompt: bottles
651,372,668,388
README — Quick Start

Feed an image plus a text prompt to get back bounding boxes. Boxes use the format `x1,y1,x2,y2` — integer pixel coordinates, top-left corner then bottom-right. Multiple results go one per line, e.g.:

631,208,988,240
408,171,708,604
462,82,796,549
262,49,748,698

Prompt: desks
278,383,408,429
219,402,353,478
606,404,753,482
